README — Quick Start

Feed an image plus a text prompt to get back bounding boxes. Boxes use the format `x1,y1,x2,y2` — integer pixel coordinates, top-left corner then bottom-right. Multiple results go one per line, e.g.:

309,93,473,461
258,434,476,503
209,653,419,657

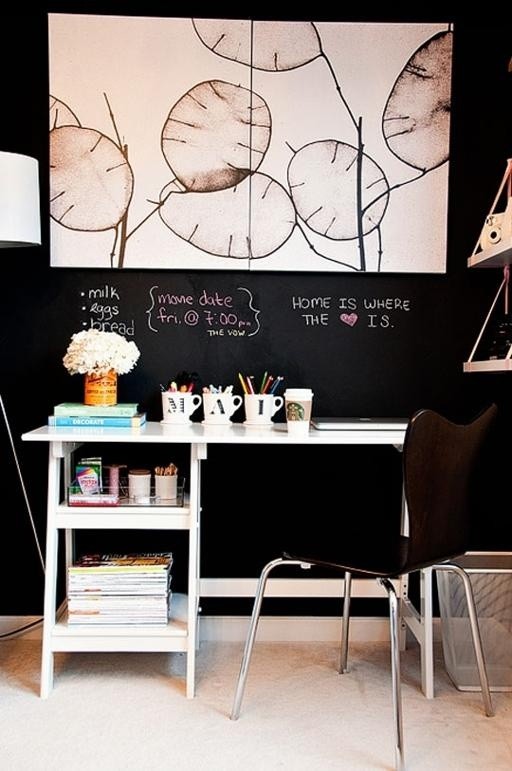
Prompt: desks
21,421,440,701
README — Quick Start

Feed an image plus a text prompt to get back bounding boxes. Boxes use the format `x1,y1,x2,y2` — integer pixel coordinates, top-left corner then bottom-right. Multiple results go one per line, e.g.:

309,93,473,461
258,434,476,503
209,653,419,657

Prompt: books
53,402,138,418
63,548,176,630
48,414,148,429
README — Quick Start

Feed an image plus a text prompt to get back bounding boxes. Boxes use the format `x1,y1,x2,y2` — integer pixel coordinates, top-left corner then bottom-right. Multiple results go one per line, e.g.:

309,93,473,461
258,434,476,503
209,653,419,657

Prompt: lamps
0,149,47,577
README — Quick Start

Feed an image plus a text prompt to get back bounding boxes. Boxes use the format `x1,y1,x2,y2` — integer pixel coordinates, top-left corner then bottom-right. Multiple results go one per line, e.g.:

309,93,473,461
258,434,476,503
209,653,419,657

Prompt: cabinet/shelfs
462,158,512,373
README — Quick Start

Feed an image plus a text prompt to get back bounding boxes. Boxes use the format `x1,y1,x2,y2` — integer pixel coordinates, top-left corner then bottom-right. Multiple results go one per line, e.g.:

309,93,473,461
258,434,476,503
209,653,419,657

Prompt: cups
129,470,178,500
160,388,313,435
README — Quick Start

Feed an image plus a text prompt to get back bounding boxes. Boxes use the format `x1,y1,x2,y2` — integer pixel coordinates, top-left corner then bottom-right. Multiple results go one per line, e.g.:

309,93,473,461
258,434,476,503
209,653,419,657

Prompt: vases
83,370,118,407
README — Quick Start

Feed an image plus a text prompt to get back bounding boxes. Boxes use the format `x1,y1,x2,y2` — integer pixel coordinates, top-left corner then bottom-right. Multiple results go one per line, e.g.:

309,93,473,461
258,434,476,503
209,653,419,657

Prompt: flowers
62,327,140,379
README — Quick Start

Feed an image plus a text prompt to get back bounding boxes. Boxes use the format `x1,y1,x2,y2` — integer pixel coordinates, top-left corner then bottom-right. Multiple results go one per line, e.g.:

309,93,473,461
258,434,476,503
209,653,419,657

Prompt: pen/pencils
154,462,179,476
201,383,235,393
239,370,285,394
157,380,194,392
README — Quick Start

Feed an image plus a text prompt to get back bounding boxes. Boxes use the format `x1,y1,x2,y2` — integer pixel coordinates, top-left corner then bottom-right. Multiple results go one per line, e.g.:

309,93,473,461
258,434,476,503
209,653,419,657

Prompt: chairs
222,401,501,771
